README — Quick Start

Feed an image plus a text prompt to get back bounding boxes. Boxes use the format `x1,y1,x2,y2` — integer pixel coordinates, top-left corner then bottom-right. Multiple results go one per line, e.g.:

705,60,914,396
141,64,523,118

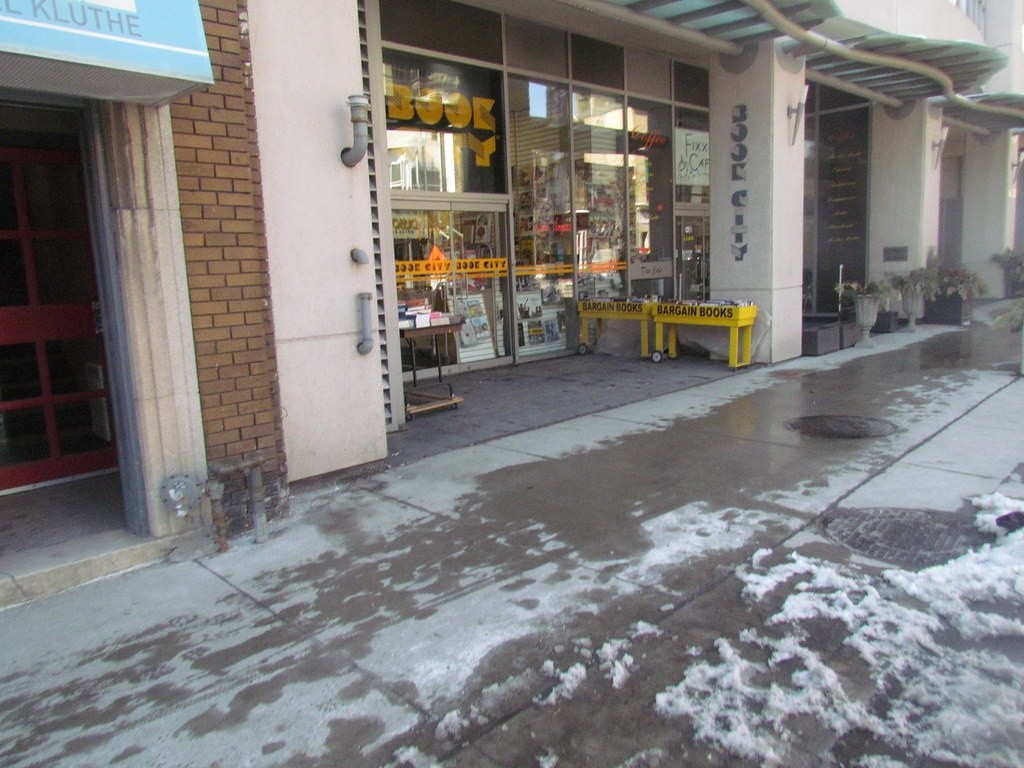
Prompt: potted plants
832,276,903,349
990,248,1024,299
889,266,941,336
924,246,990,325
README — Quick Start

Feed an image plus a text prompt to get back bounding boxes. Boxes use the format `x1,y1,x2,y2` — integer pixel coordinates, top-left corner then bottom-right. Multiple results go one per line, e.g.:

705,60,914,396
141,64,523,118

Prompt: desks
400,323,462,387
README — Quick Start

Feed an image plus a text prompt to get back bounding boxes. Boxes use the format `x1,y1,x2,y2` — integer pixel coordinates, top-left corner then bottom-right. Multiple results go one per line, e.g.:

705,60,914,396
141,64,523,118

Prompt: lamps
1011,151,1024,184
786,85,811,145
932,127,950,169
